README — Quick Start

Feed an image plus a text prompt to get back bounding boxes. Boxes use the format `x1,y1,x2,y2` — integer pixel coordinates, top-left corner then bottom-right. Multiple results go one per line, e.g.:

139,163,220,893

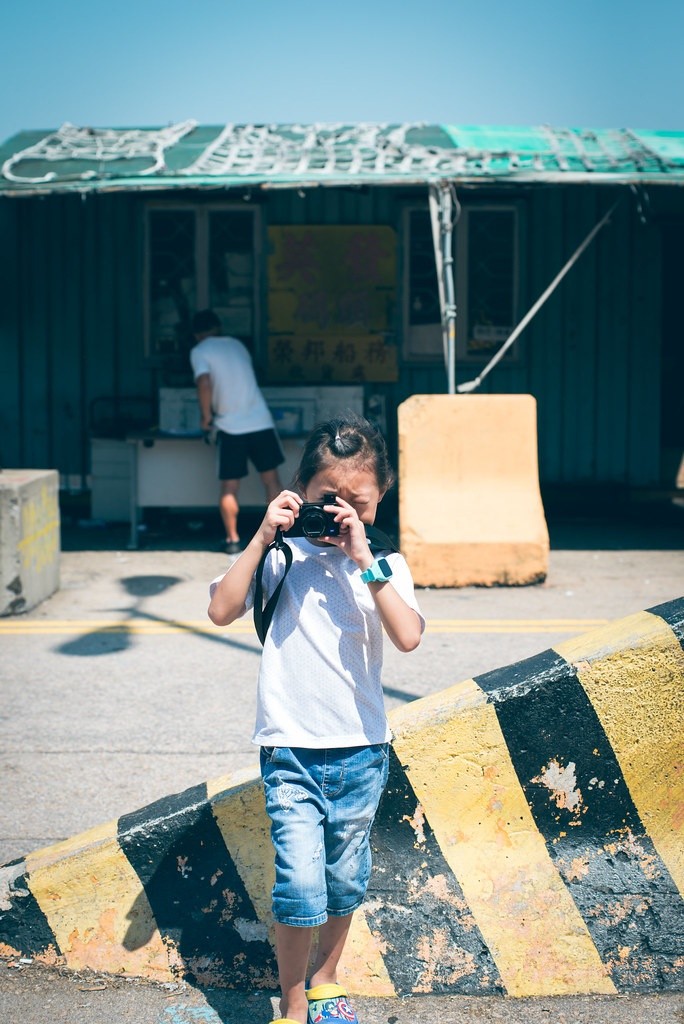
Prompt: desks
127,430,313,550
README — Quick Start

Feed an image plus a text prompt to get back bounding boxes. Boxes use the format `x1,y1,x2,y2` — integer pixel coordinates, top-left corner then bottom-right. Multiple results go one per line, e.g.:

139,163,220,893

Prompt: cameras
282,493,340,538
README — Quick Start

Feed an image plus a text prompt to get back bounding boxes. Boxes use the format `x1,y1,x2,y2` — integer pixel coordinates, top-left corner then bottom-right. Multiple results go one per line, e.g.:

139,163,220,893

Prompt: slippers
305,979,358,1024
268,1019,302,1024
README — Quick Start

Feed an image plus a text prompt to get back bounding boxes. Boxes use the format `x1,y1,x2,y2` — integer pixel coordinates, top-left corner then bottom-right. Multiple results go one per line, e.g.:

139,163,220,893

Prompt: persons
189,310,285,553
211,411,428,1024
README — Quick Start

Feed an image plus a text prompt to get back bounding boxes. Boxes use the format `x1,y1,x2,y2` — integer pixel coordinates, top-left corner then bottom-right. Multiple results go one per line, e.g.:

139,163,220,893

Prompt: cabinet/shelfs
89,433,134,523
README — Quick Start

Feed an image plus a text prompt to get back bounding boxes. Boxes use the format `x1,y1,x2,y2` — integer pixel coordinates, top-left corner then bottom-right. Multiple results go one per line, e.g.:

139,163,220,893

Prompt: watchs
360,555,393,584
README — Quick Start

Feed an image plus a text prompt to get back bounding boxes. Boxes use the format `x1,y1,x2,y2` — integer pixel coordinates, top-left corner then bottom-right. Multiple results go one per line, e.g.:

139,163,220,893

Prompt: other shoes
220,537,241,552
